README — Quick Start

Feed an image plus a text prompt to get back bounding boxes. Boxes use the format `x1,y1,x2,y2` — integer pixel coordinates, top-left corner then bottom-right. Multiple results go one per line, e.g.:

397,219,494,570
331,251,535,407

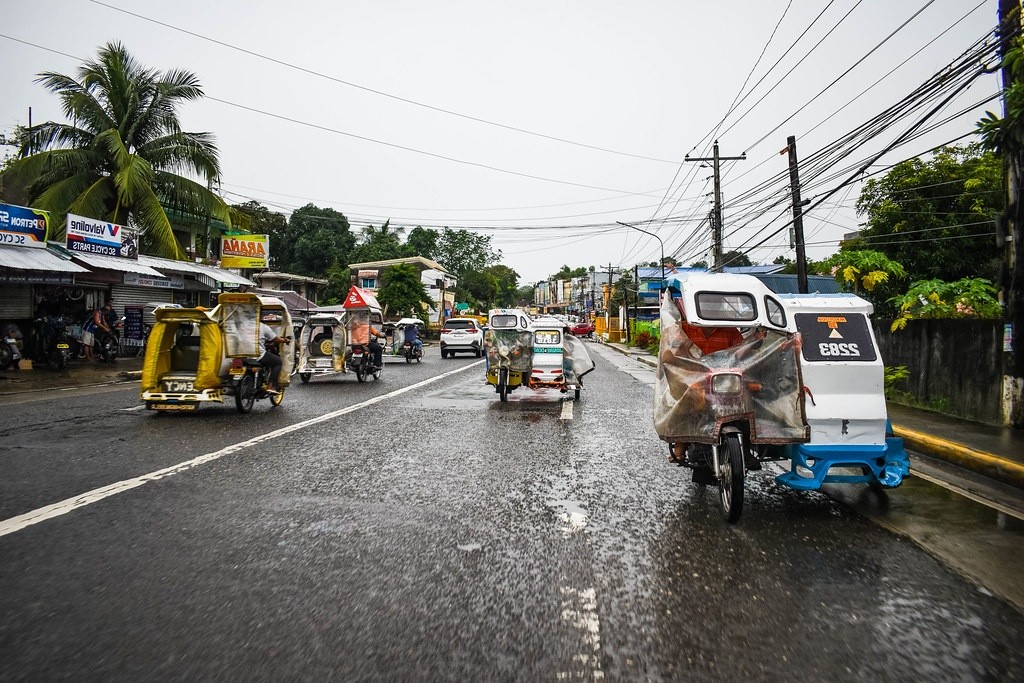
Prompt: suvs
439,317,485,359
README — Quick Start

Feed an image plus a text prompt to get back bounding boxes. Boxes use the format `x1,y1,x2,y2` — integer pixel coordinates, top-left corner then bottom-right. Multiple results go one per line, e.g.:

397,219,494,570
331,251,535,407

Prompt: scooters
0,312,153,370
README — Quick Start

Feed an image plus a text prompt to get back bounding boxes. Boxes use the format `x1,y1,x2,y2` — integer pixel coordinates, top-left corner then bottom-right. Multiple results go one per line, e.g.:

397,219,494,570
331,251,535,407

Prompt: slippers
266,387,281,395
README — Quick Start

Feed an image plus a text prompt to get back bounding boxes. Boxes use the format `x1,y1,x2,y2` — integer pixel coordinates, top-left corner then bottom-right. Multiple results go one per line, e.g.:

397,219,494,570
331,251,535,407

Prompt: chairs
307,338,333,367
158,345,200,391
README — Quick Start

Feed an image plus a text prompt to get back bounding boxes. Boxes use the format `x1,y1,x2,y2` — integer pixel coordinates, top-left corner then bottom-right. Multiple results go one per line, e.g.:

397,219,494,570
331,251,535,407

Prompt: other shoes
374,366,383,371
666,454,686,463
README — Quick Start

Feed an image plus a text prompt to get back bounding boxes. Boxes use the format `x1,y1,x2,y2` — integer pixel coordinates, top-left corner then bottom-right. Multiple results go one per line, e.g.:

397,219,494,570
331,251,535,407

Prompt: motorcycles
139,293,296,415
298,283,393,383
482,309,566,401
382,318,425,363
636,273,912,522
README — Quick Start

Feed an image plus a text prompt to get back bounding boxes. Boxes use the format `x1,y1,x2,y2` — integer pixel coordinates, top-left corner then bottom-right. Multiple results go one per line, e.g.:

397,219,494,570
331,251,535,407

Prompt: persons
82,305,111,360
237,307,290,396
661,293,766,463
101,297,120,336
405,323,425,357
488,315,523,364
564,326,583,400
351,309,386,370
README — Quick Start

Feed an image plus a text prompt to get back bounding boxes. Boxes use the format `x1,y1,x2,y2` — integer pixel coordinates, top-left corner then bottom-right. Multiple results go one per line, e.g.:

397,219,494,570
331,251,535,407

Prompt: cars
569,323,595,338
524,307,584,326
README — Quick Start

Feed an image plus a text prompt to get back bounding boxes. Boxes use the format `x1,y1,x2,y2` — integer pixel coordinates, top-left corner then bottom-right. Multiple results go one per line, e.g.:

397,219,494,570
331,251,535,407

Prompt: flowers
956,298,975,316
